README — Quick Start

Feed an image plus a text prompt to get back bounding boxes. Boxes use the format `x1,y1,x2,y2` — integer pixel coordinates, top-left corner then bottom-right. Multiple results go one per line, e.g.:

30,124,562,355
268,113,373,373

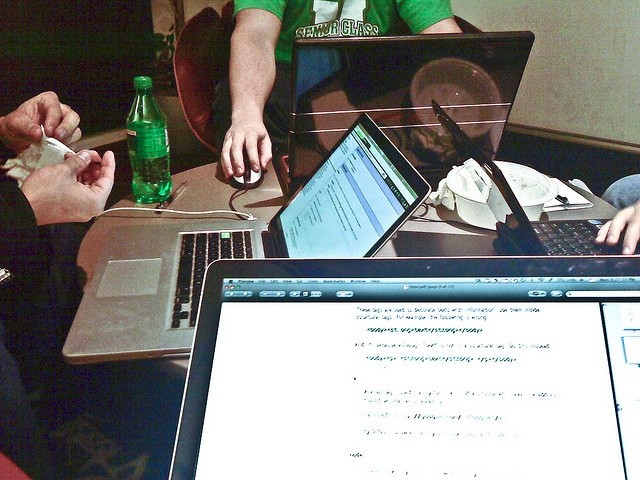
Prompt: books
543,177,593,214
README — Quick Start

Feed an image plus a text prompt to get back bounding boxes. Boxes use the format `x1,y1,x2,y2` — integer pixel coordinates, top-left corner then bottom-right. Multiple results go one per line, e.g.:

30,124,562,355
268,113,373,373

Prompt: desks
75,162,617,385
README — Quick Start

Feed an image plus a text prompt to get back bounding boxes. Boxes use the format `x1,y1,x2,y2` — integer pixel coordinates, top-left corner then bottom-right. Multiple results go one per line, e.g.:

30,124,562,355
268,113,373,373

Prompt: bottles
125,76,172,204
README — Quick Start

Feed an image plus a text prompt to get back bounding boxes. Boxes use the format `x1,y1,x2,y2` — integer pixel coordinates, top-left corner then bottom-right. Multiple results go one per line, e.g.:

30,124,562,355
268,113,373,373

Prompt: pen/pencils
154,180,188,214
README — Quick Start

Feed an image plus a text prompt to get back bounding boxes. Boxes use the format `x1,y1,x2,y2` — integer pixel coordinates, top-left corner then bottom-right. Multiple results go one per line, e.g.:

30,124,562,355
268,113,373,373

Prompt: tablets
163,255,640,476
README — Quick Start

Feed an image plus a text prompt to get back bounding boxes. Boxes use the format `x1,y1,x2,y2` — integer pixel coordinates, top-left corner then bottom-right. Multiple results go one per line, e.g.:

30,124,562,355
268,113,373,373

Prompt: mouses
229,158,265,188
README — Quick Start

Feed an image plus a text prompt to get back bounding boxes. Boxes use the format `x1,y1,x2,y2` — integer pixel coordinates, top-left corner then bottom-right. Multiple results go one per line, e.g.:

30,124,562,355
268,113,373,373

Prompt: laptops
430,98,640,255
273,30,534,199
62,110,431,365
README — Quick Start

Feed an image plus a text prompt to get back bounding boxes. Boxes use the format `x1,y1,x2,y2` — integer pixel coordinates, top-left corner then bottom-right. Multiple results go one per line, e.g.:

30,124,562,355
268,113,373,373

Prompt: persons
219,1,462,179
589,170,640,257
0,90,116,283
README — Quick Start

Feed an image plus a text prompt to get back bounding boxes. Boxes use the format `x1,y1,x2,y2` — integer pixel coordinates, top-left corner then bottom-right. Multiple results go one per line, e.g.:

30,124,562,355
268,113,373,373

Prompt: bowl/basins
408,58,505,150
446,160,556,231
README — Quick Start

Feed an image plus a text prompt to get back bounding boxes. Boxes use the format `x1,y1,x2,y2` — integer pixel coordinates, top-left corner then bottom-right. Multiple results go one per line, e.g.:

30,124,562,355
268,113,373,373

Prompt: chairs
174,2,236,157
